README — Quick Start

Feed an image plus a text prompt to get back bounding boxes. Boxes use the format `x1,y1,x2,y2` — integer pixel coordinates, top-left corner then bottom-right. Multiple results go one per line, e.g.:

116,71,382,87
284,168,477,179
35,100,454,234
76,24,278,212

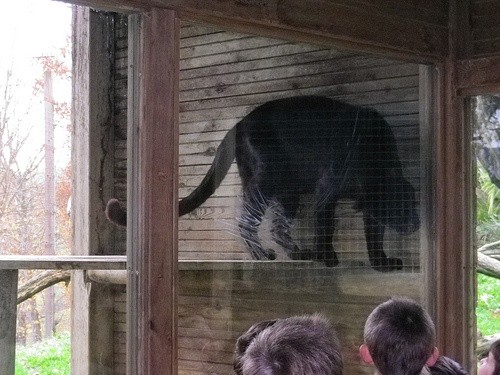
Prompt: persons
240,312,345,375
476,339,500,375
359,296,439,375
430,353,466,375
232,318,277,375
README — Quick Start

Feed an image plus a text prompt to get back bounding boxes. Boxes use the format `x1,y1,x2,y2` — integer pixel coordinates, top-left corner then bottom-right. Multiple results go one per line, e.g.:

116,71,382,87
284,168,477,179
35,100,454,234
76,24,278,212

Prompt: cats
105,92,422,272
